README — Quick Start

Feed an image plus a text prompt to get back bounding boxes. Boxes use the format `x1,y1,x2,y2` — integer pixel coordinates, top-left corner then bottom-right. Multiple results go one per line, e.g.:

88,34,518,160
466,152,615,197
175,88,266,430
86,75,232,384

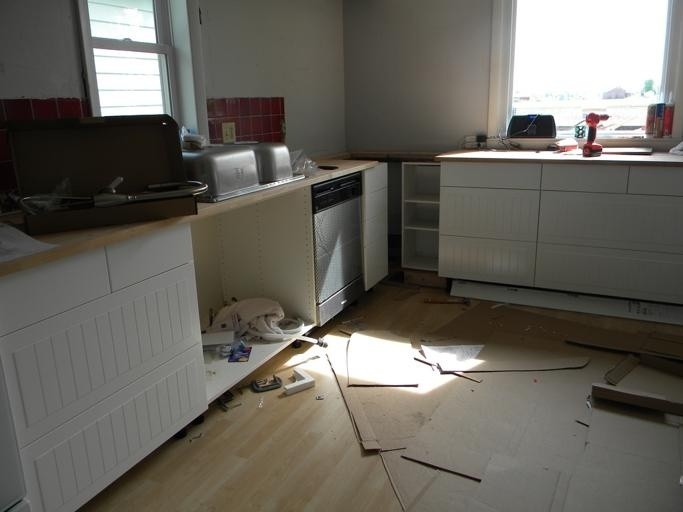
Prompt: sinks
182,142,307,204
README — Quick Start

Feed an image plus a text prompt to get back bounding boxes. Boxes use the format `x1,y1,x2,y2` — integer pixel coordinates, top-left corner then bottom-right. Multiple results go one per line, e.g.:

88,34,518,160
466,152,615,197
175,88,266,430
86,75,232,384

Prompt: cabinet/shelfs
534,146,682,308
0,158,388,512
435,146,542,292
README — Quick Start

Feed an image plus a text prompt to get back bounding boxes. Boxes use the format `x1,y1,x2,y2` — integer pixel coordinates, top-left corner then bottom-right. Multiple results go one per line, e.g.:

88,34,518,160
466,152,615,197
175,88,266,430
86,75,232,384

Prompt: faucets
89,175,210,209
